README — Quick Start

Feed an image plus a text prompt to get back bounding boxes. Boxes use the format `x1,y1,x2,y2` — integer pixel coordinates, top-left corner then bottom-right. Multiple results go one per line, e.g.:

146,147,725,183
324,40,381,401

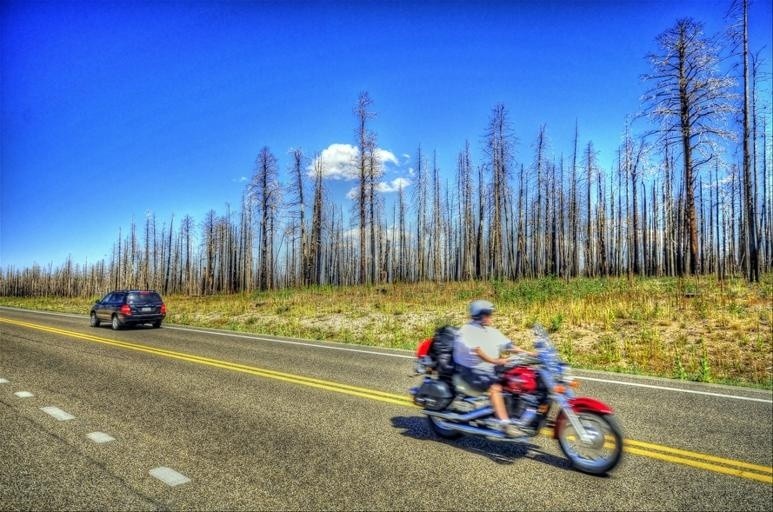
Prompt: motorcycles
410,325,623,474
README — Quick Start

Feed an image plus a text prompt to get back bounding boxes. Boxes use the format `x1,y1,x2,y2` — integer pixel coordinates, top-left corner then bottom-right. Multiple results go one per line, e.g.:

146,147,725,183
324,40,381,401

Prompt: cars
90,289,167,330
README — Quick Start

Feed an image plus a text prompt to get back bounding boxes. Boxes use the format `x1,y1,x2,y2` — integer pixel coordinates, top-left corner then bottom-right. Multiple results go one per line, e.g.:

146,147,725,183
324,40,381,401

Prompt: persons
446,298,538,440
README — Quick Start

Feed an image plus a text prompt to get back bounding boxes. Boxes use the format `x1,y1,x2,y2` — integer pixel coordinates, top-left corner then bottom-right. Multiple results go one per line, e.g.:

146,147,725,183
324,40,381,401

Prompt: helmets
470,300,495,316
